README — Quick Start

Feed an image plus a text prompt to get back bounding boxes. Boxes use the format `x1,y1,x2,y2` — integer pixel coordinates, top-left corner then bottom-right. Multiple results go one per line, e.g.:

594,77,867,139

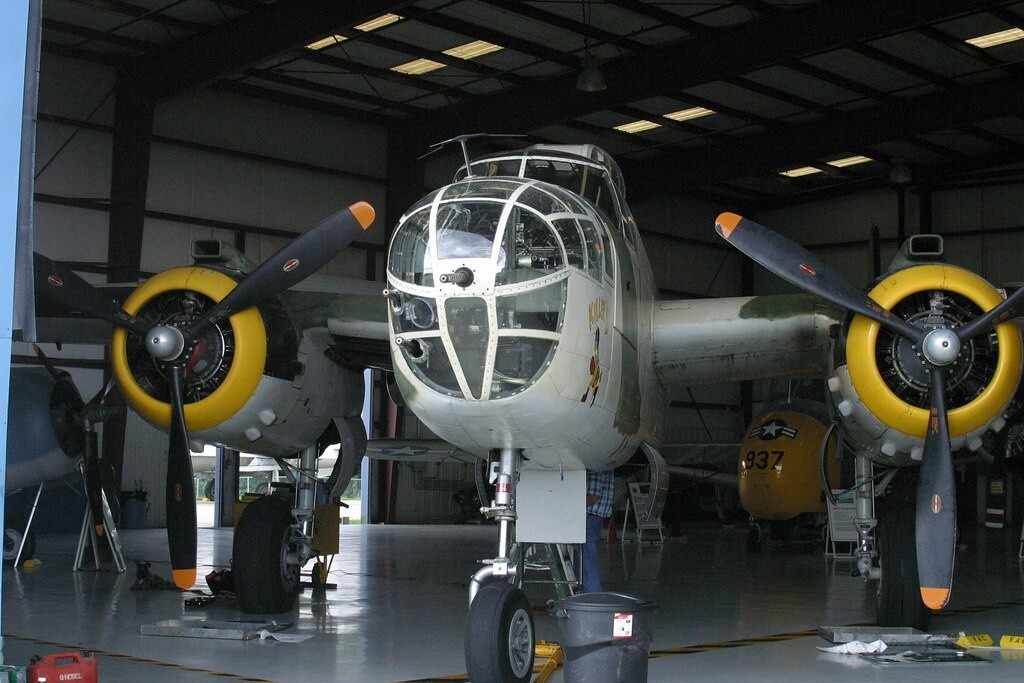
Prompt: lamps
575,0,607,92
888,137,912,182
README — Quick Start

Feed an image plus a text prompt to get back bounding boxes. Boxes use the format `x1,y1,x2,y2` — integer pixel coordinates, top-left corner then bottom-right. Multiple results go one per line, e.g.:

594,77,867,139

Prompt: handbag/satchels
206,569,236,596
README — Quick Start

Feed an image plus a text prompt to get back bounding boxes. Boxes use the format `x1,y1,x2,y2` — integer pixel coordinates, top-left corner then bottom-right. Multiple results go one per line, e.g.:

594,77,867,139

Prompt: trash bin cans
556,593,656,683
121,490,151,528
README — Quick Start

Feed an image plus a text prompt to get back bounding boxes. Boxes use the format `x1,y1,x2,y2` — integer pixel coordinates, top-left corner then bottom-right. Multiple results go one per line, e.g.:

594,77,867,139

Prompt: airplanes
3,130,1024,683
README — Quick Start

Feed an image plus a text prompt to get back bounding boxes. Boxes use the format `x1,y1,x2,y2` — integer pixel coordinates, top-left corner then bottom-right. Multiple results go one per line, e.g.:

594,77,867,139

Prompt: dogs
130,561,170,589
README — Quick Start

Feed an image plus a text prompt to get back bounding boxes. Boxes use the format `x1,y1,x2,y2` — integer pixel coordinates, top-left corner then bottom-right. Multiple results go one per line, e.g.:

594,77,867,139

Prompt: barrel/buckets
120,491,149,529
544,590,653,683
26,650,98,683
0,664,25,683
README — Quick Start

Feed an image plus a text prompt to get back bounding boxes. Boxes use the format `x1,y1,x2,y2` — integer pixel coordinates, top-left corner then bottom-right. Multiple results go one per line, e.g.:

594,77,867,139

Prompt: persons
584,470,614,592
